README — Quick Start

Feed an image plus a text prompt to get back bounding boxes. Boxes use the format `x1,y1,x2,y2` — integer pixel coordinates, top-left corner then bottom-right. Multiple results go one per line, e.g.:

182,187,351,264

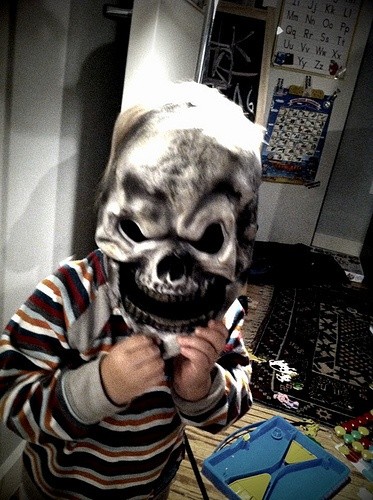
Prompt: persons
0,81,268,500
